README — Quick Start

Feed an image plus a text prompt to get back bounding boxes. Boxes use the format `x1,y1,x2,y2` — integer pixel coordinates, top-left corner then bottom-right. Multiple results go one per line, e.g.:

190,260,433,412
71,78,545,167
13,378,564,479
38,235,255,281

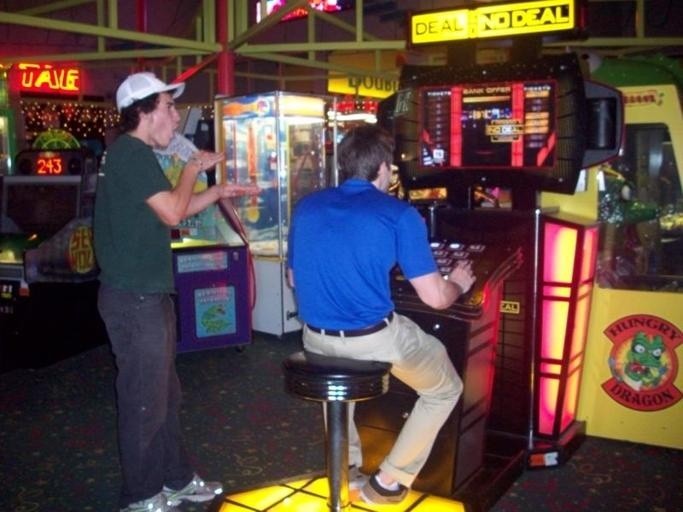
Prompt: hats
115,72,186,115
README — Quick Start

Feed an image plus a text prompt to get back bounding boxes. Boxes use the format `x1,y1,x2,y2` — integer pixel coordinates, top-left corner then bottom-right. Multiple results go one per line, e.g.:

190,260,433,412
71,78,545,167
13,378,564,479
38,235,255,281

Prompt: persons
278,121,479,507
87,68,261,512
657,154,674,208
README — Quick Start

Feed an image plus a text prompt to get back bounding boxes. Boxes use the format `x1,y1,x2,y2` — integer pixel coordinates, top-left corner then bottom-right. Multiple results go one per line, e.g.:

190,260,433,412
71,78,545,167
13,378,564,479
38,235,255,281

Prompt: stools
282,350,393,512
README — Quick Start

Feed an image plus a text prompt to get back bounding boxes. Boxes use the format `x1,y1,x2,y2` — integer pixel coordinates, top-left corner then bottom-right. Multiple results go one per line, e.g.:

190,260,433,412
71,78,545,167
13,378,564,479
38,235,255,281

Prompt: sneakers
348,464,369,491
119,492,180,512
359,468,408,506
161,471,224,508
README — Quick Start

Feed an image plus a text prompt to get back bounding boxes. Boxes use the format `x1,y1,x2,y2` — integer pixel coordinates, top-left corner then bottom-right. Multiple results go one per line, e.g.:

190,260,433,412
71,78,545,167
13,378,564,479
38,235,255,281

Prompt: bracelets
191,154,204,174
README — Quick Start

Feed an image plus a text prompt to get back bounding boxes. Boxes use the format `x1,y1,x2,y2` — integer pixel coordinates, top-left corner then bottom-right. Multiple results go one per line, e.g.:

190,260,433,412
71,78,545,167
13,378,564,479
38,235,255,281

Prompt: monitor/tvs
255,0,346,26
418,81,560,172
2,176,82,235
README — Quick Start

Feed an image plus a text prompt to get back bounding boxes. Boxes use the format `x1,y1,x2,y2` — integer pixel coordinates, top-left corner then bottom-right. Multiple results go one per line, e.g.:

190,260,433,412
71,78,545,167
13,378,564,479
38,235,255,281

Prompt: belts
303,310,396,336
